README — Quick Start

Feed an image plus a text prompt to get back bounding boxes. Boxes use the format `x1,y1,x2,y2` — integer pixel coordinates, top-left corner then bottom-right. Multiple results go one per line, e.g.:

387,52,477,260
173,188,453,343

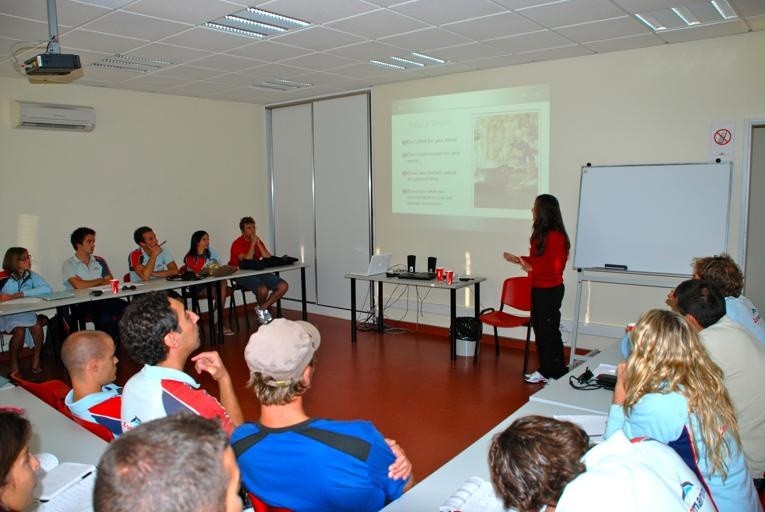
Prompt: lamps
471,275,533,381
11,368,69,408
244,490,295,512
55,398,116,444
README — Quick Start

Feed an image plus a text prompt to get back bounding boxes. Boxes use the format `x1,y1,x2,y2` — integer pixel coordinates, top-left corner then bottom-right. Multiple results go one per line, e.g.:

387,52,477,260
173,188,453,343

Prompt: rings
159,240,167,247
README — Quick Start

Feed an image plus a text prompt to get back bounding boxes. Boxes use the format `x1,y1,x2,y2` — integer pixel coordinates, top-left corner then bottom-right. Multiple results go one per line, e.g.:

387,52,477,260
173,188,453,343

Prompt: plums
25,53,80,75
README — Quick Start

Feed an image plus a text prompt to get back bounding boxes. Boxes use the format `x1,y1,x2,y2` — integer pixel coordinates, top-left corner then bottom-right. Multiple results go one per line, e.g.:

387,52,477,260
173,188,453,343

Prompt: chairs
254,304,272,322
256,309,268,324
523,371,549,383
544,377,557,387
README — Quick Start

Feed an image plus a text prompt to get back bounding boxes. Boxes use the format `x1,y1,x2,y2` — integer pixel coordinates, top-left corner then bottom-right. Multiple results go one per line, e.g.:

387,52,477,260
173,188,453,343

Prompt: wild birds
348,254,392,276
398,272,434,279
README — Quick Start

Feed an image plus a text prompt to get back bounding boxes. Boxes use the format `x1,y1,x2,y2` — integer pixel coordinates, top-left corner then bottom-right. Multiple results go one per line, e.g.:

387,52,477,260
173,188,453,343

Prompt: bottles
244,316,323,386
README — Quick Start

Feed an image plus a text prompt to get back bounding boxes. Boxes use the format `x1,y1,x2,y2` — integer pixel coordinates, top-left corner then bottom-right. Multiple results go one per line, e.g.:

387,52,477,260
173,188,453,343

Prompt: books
554,414,608,442
31,452,96,500
442,477,548,511
592,364,617,377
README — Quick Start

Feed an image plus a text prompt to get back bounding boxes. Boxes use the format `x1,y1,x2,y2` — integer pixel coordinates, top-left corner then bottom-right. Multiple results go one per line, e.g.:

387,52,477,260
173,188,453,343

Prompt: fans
572,161,733,278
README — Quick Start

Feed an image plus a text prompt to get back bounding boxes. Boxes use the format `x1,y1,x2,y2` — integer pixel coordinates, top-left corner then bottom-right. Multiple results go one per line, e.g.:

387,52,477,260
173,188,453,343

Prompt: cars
445,271,454,285
110,277,120,295
628,322,636,331
436,267,445,282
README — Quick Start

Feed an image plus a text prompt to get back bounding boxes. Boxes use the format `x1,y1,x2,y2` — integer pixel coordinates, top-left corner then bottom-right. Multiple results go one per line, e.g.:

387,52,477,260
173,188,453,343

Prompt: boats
428,257,436,272
407,255,416,272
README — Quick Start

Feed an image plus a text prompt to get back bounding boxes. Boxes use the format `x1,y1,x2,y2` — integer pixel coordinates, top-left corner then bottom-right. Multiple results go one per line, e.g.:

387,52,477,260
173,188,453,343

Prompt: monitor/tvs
592,364,617,377
442,477,548,511
554,414,608,442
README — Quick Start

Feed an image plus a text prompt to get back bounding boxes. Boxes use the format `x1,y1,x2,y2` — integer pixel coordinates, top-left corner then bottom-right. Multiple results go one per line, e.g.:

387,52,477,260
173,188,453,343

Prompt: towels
455,317,481,356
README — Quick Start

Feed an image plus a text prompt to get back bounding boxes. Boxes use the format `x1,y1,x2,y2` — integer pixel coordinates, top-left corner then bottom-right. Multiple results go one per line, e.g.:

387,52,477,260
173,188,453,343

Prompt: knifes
8,97,97,135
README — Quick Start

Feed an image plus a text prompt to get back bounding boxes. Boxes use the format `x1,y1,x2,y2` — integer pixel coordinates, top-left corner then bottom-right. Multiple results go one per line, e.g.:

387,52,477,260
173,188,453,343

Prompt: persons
129,226,179,296
0,247,53,377
62,330,124,440
693,253,765,342
93,413,242,512
184,230,234,335
63,227,128,354
228,318,412,512
488,416,718,512
120,292,245,440
0,411,39,512
231,218,288,323
502,194,570,387
666,278,765,493
604,309,765,512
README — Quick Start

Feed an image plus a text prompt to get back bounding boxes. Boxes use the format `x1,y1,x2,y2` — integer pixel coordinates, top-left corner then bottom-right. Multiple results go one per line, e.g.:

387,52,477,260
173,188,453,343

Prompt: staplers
604,264,628,271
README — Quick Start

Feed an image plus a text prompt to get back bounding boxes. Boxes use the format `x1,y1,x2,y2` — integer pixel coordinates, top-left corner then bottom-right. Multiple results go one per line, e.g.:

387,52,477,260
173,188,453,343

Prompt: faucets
214,326,235,336
32,364,43,375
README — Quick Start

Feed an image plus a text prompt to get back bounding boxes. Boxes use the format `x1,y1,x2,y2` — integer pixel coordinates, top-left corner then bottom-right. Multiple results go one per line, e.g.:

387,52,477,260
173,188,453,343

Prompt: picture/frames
0,377,13,392
343,270,486,364
0,380,111,512
374,398,607,511
529,338,624,414
